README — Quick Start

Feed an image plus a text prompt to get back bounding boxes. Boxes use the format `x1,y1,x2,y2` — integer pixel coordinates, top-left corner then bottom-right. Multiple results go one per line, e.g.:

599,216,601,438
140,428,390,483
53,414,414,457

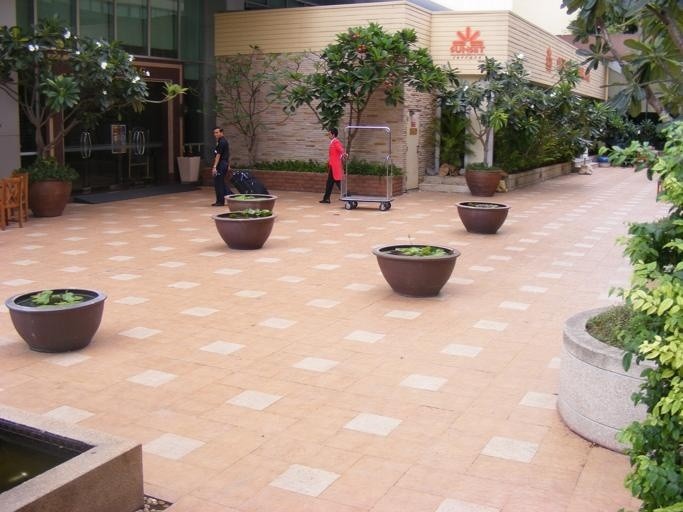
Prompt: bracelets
213,166,217,168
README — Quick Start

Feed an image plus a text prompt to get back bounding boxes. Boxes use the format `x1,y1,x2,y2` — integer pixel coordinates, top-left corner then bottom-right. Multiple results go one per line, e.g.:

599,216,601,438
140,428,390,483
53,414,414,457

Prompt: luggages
229,168,269,194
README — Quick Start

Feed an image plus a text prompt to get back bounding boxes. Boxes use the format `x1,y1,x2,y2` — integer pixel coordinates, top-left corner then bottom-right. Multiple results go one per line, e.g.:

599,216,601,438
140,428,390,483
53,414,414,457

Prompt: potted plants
4,286,107,353
431,56,546,232
10,156,79,218
213,192,277,248
373,244,460,297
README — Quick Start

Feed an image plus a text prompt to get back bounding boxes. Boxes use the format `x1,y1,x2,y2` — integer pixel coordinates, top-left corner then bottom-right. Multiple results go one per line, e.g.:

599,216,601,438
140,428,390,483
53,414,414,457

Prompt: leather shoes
212,202,224,206
319,198,330,203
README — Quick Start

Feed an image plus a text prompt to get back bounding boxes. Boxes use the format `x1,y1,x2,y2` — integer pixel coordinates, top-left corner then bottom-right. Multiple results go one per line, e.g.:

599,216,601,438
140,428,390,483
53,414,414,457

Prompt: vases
174,155,200,184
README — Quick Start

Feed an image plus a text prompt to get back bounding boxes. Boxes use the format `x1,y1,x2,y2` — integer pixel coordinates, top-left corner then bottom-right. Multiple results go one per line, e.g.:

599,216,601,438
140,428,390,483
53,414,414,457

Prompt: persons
211,126,231,206
319,128,351,203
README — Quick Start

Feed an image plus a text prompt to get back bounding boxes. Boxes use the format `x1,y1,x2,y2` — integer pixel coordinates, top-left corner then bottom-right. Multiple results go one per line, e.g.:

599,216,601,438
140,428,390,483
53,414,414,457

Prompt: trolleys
338,124,397,211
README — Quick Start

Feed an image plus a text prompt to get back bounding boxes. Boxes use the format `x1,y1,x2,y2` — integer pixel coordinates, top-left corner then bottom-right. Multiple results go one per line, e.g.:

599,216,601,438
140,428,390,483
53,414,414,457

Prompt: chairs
0,172,29,230
